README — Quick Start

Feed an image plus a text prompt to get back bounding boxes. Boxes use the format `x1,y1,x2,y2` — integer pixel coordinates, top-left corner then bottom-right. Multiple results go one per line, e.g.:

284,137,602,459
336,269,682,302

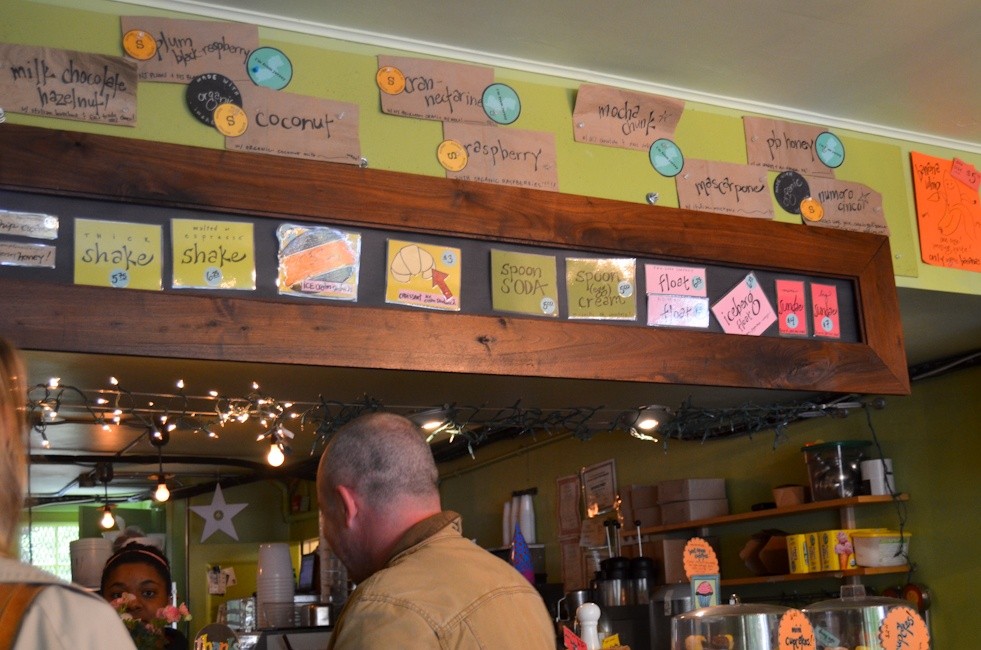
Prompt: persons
99,542,182,649
0,335,136,650
315,413,557,649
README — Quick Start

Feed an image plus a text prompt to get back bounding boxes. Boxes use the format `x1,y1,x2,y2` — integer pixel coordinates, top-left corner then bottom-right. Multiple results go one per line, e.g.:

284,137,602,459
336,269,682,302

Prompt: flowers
107,592,193,650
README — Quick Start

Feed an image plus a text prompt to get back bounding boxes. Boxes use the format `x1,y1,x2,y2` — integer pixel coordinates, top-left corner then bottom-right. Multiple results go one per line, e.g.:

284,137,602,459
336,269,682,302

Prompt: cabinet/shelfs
621,492,920,599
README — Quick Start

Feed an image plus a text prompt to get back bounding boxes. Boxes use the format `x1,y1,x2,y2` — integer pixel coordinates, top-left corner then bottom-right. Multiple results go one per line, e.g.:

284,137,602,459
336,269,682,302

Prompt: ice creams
834,532,853,570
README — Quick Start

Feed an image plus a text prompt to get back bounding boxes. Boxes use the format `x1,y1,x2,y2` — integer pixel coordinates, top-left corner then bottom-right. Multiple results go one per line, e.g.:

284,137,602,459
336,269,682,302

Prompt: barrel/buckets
257,542,295,628
69,539,113,588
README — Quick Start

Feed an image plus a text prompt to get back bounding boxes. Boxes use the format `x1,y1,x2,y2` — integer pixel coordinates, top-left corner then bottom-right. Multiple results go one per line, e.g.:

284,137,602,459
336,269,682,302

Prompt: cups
502,494,536,545
558,621,576,650
558,589,593,622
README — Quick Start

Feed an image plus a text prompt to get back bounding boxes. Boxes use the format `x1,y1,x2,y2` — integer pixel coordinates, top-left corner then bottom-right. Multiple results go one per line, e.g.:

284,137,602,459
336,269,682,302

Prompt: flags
515,522,534,585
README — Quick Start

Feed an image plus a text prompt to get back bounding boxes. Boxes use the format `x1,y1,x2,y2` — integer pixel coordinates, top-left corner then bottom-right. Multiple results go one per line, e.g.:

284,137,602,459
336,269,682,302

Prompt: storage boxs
784,527,914,575
620,544,632,558
798,439,875,504
615,476,731,530
632,539,691,585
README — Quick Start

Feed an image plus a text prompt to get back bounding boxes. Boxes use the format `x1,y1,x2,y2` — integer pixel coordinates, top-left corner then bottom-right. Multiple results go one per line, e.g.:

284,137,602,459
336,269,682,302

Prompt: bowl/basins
263,603,304,627
773,486,805,509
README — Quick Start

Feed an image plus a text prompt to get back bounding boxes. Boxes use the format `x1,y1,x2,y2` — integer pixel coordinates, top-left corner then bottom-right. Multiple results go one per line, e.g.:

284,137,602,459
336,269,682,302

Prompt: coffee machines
629,557,659,618
589,557,637,621
